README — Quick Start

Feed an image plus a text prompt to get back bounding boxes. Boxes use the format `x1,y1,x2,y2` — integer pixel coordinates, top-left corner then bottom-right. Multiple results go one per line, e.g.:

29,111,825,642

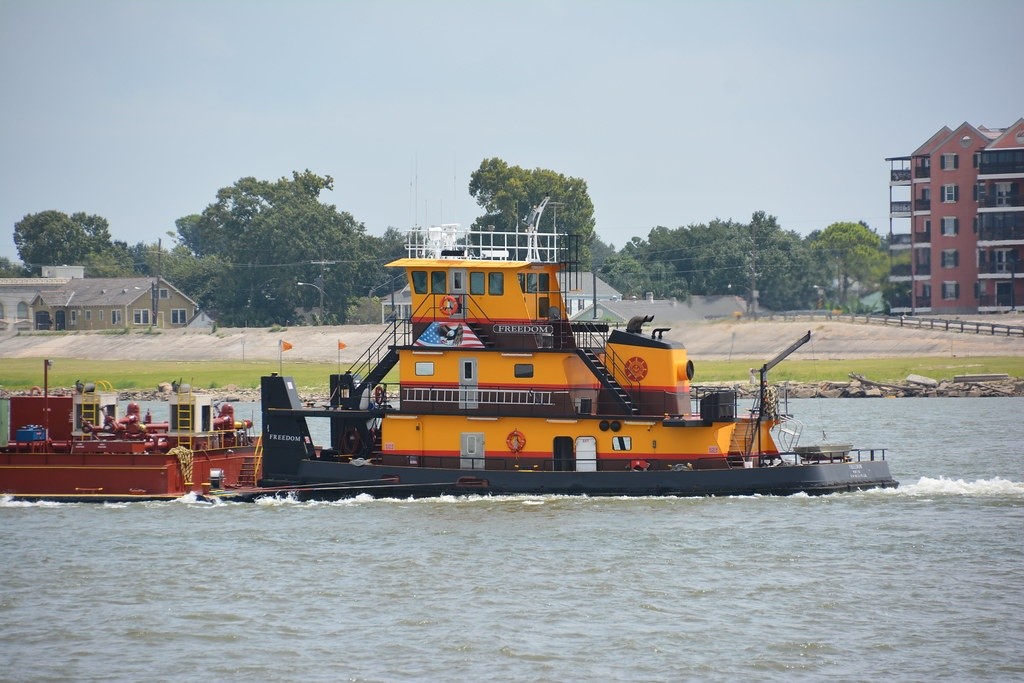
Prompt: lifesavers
507,431,526,452
440,295,458,316
375,386,384,404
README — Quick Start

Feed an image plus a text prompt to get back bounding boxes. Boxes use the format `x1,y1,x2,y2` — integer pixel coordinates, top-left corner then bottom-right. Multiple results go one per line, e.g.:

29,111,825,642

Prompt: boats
238,172,901,504
0,358,322,504
